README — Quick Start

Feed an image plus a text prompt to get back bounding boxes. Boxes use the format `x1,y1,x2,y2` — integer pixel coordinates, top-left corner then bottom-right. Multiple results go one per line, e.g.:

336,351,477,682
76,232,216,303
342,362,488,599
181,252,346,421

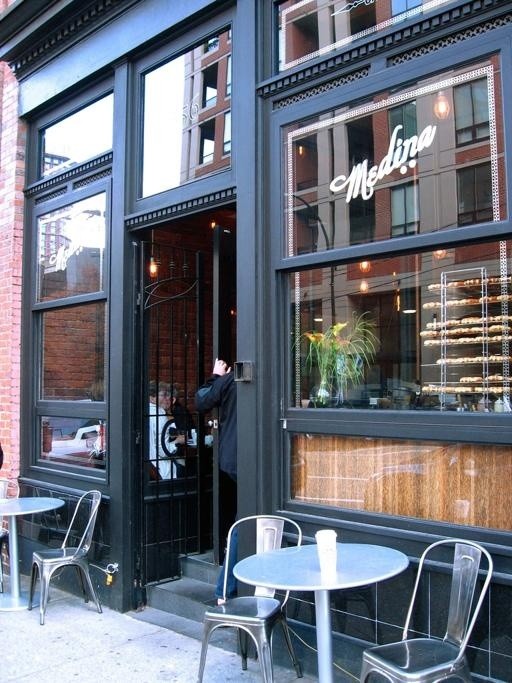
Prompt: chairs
0,477,104,624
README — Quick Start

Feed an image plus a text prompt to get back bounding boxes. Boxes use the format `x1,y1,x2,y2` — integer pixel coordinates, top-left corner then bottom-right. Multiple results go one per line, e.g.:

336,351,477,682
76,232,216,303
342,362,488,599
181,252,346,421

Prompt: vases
308,382,340,408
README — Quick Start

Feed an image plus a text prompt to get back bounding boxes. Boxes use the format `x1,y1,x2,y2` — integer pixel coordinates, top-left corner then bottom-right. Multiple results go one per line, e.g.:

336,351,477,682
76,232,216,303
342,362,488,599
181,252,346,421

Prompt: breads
419,277,512,393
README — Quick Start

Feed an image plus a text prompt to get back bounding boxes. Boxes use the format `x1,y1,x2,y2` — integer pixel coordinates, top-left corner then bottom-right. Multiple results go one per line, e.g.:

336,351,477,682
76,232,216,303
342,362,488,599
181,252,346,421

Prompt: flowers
294,310,382,406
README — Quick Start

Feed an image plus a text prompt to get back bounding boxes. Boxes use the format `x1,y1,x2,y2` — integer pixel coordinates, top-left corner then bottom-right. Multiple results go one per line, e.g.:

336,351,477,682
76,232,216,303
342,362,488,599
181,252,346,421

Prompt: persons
148,380,187,478
194,357,238,600
162,382,195,430
91,373,105,401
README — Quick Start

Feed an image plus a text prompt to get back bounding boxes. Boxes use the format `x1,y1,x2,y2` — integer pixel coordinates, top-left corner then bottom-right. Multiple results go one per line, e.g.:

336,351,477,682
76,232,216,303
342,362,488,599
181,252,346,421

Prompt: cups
320,561,338,588
314,529,338,560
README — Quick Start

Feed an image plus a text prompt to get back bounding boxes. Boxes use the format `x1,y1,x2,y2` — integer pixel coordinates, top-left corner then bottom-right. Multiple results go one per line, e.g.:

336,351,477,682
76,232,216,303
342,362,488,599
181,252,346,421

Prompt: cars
39,394,107,466
363,443,511,533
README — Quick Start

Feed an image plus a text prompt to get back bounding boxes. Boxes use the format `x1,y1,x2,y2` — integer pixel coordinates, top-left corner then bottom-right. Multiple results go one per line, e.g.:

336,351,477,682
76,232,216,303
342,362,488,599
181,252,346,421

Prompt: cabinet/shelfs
419,266,512,410
